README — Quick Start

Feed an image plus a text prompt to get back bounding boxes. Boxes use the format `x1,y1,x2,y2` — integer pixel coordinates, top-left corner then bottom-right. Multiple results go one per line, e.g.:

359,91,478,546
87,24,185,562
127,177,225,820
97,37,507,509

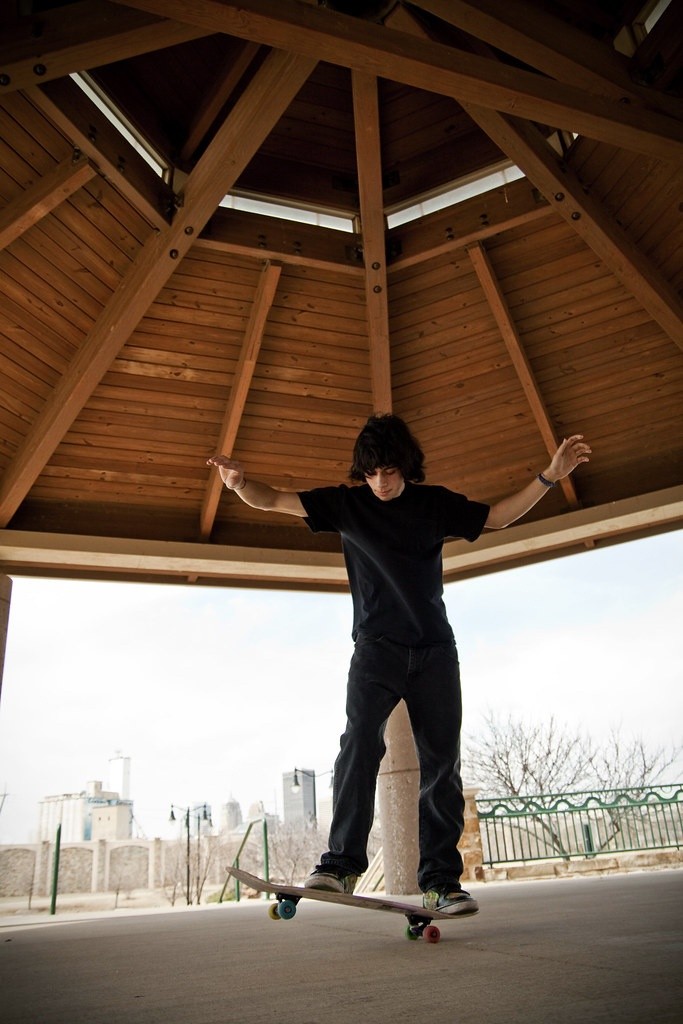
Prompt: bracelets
537,472,558,488
235,478,246,493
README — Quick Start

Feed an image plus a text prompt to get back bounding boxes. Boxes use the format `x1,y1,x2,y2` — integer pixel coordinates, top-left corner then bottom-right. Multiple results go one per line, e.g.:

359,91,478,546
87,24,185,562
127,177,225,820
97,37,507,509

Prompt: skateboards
225,866,480,944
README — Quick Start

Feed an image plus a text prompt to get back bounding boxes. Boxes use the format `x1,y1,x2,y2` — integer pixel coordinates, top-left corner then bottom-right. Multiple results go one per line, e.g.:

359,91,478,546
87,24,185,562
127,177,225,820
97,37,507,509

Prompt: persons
204,414,597,915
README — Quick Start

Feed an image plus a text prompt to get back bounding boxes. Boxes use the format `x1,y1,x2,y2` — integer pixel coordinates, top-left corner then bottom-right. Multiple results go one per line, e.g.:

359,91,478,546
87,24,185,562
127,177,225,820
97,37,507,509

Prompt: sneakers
305,863,358,895
423,883,479,914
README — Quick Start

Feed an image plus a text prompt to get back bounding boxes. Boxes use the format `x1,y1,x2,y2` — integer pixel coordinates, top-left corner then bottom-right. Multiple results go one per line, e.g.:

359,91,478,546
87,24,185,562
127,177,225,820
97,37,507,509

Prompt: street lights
167,802,209,904
